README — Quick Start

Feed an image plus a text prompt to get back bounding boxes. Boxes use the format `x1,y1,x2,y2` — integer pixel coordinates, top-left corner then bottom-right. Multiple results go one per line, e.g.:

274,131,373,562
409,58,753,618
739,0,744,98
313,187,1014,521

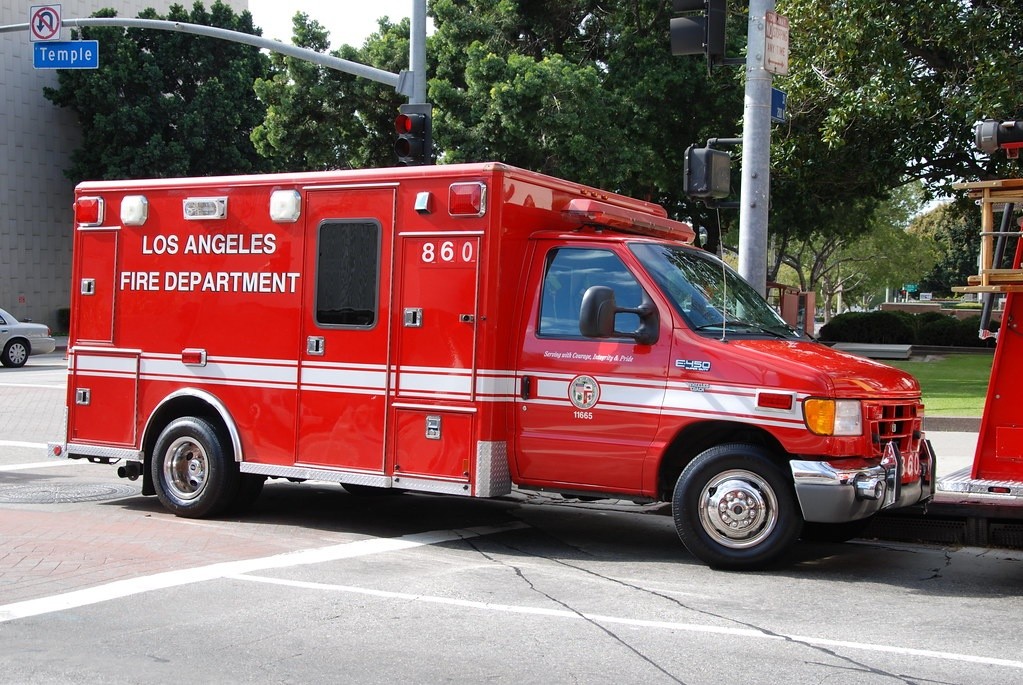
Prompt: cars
0,309,54,368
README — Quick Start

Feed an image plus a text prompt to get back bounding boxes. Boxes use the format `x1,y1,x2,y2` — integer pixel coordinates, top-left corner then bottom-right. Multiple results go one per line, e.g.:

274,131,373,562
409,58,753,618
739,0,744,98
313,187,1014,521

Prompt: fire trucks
939,118,1023,503
43,161,939,571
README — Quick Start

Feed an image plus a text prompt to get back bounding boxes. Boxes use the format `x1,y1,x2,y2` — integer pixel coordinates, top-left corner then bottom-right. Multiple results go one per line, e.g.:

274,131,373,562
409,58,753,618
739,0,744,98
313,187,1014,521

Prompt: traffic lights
669,0,726,74
899,290,907,295
395,104,432,165
